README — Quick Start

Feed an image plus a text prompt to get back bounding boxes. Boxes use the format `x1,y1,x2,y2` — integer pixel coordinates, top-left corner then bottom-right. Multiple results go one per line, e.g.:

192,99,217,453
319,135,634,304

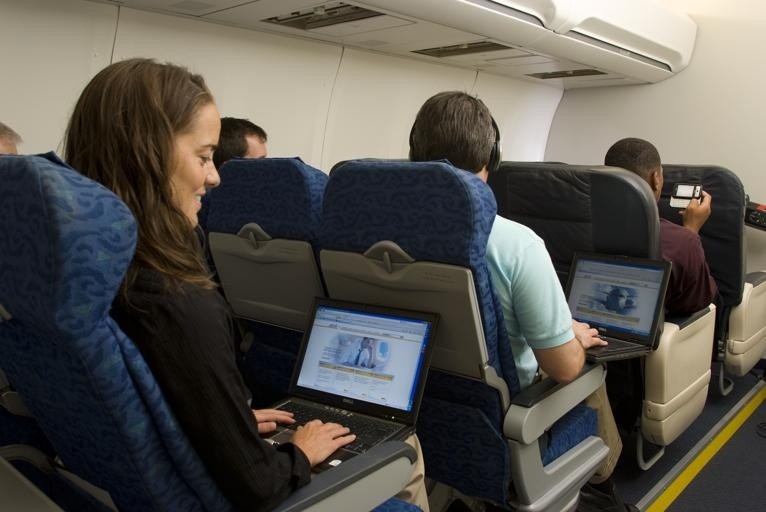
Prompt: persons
338,337,370,367
0,121,22,157
406,89,656,512
211,117,268,172
604,136,720,325
46,58,432,512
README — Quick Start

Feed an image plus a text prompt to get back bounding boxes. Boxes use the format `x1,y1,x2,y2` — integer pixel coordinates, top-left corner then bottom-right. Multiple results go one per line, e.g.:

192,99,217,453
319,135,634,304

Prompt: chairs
0,154,766,512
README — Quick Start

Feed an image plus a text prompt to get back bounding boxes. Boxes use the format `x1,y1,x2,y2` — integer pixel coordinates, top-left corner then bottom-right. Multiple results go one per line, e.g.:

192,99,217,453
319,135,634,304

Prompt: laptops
258,296,441,474
565,249,673,363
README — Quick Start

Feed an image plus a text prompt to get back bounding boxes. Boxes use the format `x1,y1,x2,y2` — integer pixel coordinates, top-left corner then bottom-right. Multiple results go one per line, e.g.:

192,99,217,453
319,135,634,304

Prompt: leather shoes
579,480,641,512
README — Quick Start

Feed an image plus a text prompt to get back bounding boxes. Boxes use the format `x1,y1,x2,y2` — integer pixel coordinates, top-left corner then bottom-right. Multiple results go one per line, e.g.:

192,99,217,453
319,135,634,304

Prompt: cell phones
670,182,704,208
